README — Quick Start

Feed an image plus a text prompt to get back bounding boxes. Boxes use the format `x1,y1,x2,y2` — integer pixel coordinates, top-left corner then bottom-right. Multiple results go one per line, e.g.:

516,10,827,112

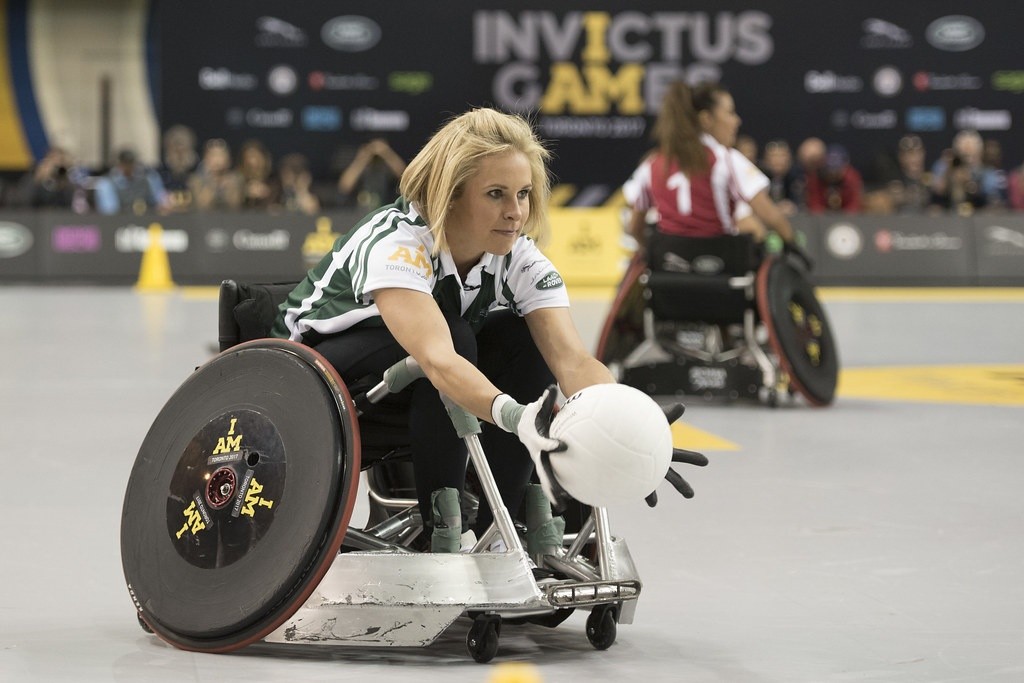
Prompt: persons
283,107,708,587
619,77,816,411
15,90,1024,258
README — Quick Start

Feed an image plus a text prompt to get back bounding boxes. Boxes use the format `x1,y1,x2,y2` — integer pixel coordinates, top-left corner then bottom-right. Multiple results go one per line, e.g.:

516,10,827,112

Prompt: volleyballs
548,383,674,510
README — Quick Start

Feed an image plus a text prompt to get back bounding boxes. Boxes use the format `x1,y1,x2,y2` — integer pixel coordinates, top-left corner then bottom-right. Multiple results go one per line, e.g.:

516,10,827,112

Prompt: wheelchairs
596,212,838,406
115,276,642,665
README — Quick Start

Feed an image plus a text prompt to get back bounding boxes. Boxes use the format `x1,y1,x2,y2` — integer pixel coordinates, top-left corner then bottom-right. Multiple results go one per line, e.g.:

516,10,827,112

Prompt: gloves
491,384,567,506
645,403,709,509
